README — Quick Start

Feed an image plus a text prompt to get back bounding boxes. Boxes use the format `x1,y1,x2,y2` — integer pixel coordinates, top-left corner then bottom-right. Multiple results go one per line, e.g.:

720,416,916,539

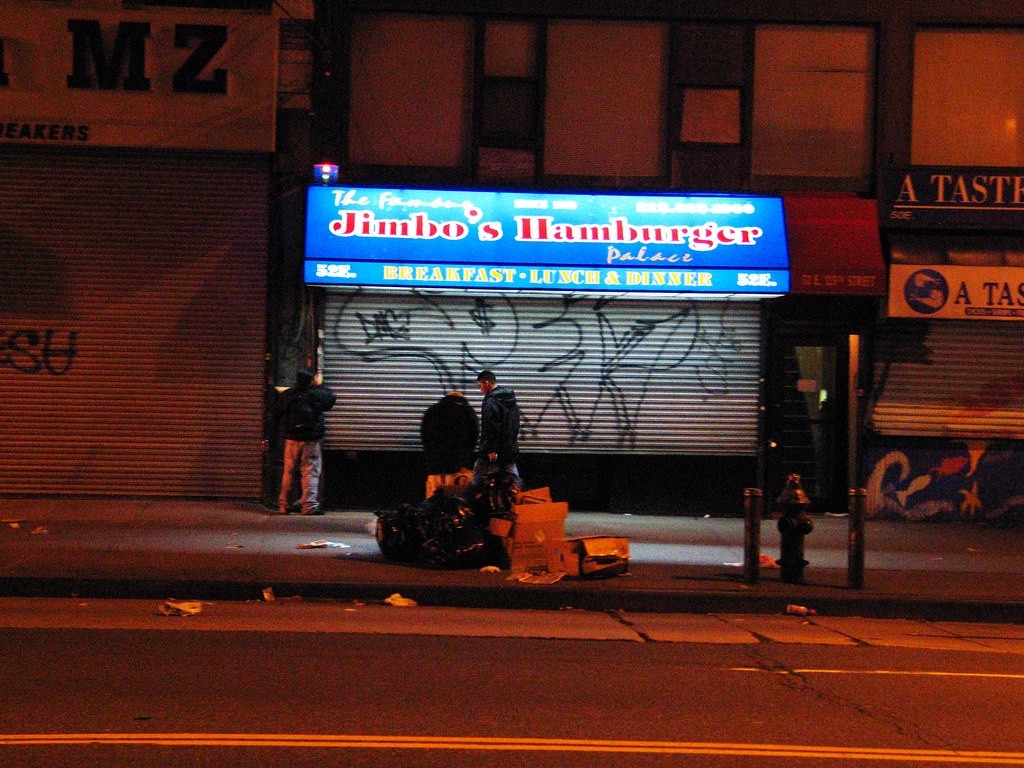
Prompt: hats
446,391,468,404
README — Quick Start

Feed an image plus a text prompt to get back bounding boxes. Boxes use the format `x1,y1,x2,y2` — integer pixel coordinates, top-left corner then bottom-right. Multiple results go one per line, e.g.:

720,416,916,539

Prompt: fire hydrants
777,473,808,586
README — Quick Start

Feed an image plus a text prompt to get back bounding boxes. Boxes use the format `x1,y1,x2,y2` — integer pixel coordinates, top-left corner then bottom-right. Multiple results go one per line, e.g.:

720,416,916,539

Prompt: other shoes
302,507,325,515
270,510,290,517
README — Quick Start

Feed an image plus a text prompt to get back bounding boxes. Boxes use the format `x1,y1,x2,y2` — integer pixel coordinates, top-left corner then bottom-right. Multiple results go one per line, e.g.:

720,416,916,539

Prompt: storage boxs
491,487,631,579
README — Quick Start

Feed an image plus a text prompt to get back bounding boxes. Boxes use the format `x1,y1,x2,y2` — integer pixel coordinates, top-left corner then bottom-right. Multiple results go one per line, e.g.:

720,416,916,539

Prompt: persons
268,366,336,515
422,392,479,500
472,370,520,485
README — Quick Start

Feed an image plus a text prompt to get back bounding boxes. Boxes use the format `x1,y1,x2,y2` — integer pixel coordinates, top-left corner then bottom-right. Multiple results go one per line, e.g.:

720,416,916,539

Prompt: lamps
313,159,340,183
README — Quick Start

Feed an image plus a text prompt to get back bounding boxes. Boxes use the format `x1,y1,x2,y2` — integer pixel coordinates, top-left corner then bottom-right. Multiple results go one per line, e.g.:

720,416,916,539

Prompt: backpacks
287,391,315,433
